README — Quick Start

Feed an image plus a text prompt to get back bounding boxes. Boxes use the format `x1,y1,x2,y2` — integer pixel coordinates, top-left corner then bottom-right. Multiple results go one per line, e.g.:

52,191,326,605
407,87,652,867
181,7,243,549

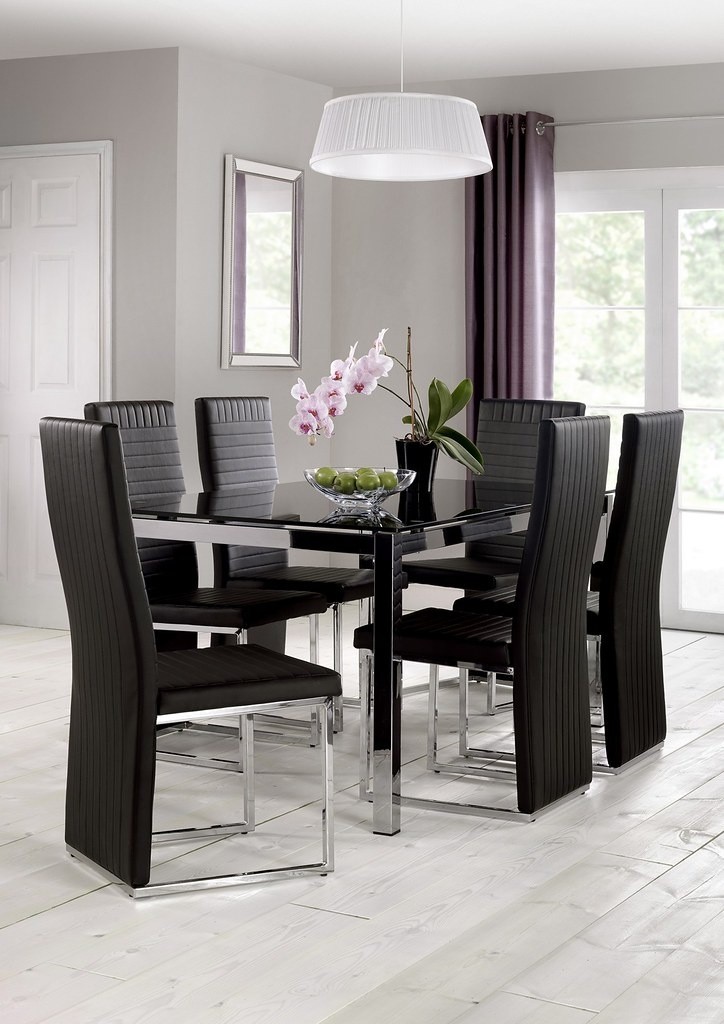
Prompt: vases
394,439,439,491
397,492,437,525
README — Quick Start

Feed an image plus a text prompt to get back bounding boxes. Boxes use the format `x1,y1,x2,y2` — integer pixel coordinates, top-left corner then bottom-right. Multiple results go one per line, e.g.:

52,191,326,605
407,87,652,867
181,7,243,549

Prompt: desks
130,481,618,836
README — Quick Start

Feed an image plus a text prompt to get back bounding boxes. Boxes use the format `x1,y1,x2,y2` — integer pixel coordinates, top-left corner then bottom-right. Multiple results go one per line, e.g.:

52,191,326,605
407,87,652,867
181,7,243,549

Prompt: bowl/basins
304,467,417,507
317,507,403,528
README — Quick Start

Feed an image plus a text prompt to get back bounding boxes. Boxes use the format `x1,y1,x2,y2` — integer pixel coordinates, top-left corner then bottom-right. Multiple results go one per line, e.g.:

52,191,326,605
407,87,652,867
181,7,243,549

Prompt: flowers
288,327,484,475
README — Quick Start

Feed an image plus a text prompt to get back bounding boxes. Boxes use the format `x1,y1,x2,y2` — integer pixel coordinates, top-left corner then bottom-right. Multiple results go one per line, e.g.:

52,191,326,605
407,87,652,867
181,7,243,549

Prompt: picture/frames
222,153,305,370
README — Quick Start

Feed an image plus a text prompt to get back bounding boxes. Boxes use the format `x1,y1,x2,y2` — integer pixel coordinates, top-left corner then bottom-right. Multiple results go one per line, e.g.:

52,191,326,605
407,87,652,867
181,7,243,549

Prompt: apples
315,467,398,495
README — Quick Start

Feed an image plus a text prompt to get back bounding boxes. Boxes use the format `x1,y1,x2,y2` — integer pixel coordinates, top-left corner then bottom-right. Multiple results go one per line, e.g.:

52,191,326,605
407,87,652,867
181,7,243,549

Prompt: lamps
308,1,494,182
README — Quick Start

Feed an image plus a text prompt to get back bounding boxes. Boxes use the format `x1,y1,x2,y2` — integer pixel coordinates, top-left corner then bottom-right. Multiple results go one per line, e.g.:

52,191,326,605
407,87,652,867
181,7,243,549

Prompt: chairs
84,397,408,771
40,417,343,900
352,398,687,822
